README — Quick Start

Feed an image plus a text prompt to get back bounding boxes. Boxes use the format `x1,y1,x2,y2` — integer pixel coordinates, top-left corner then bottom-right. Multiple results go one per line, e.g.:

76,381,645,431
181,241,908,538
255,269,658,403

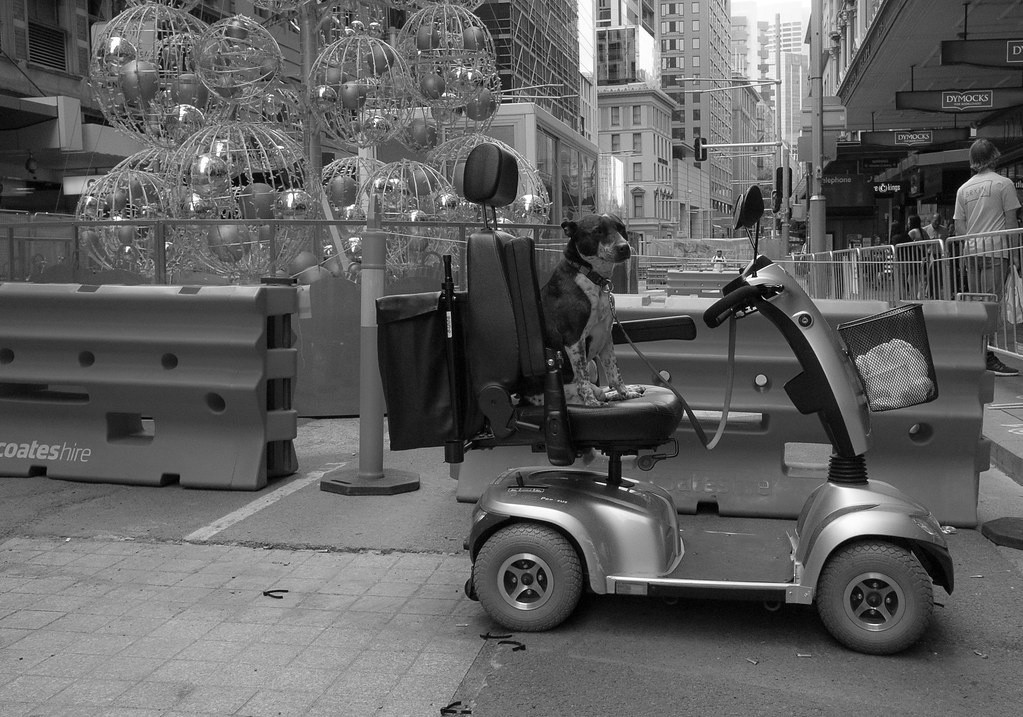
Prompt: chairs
463,144,697,464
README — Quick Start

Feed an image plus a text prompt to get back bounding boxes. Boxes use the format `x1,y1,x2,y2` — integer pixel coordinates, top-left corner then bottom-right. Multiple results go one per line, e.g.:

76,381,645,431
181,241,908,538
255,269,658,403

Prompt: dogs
521,211,646,407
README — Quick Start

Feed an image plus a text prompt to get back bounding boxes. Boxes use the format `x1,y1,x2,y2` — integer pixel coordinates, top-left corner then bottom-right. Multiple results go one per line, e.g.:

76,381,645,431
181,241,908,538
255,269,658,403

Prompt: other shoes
987,351,1020,375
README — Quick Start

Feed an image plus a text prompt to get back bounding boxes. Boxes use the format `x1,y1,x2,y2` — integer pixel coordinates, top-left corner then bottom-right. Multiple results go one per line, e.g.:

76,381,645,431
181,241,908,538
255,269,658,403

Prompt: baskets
837,304,939,412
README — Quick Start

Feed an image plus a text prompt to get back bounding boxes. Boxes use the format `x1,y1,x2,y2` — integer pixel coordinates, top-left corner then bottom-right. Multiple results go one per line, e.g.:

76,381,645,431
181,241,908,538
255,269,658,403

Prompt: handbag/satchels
1004,265,1023,324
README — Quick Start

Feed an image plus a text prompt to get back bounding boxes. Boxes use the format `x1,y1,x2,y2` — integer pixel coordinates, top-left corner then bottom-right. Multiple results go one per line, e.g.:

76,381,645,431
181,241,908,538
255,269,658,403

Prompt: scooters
435,142,1002,657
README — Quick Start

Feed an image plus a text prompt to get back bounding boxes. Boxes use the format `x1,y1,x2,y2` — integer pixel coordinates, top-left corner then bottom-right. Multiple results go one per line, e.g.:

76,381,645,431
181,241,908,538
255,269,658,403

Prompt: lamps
26,149,39,174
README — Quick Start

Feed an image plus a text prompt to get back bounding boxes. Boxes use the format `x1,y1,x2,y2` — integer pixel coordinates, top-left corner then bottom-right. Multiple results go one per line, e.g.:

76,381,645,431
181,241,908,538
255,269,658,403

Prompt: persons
711,250,727,269
891,220,921,292
907,215,930,241
952,139,1022,375
923,213,948,255
